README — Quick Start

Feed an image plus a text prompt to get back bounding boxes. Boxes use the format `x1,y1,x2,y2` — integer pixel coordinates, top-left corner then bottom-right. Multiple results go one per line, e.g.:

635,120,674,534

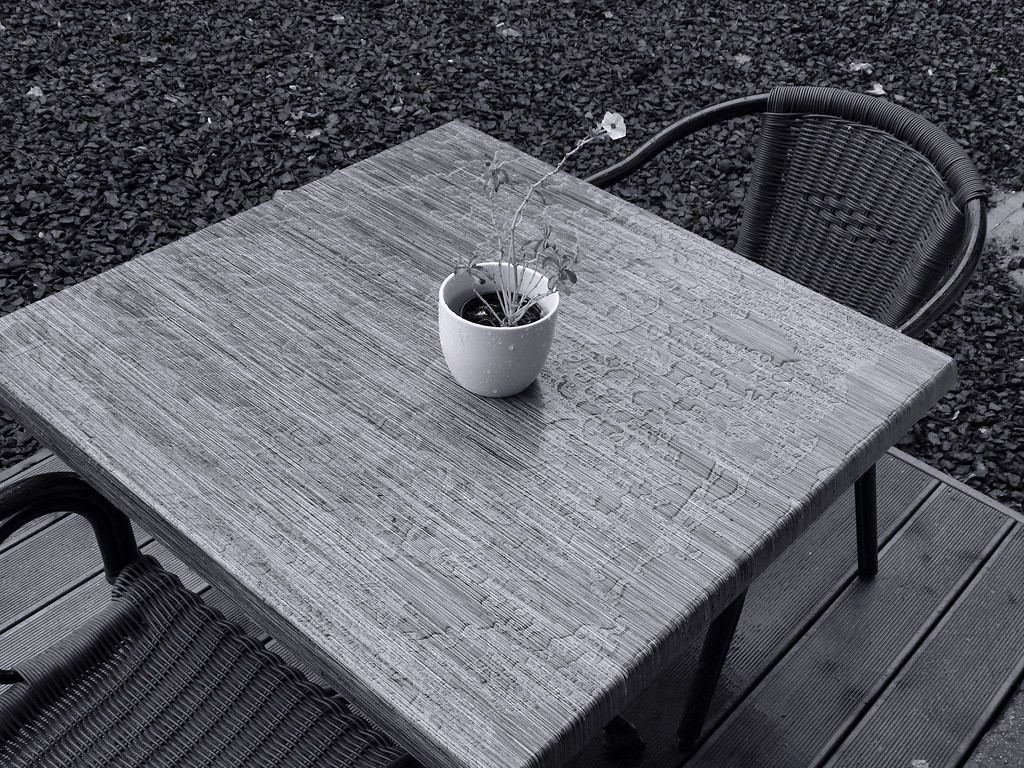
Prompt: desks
0,120,961,766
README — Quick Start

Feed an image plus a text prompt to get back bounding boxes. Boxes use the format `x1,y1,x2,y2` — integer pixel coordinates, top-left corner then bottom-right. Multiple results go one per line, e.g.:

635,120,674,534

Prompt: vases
438,263,560,400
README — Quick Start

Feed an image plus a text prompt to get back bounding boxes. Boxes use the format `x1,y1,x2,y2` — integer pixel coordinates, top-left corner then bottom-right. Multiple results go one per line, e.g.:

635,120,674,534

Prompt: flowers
448,104,630,330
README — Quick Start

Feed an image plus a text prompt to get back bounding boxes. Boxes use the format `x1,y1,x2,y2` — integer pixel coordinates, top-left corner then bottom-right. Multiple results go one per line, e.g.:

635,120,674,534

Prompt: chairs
579,88,992,760
1,470,425,768
